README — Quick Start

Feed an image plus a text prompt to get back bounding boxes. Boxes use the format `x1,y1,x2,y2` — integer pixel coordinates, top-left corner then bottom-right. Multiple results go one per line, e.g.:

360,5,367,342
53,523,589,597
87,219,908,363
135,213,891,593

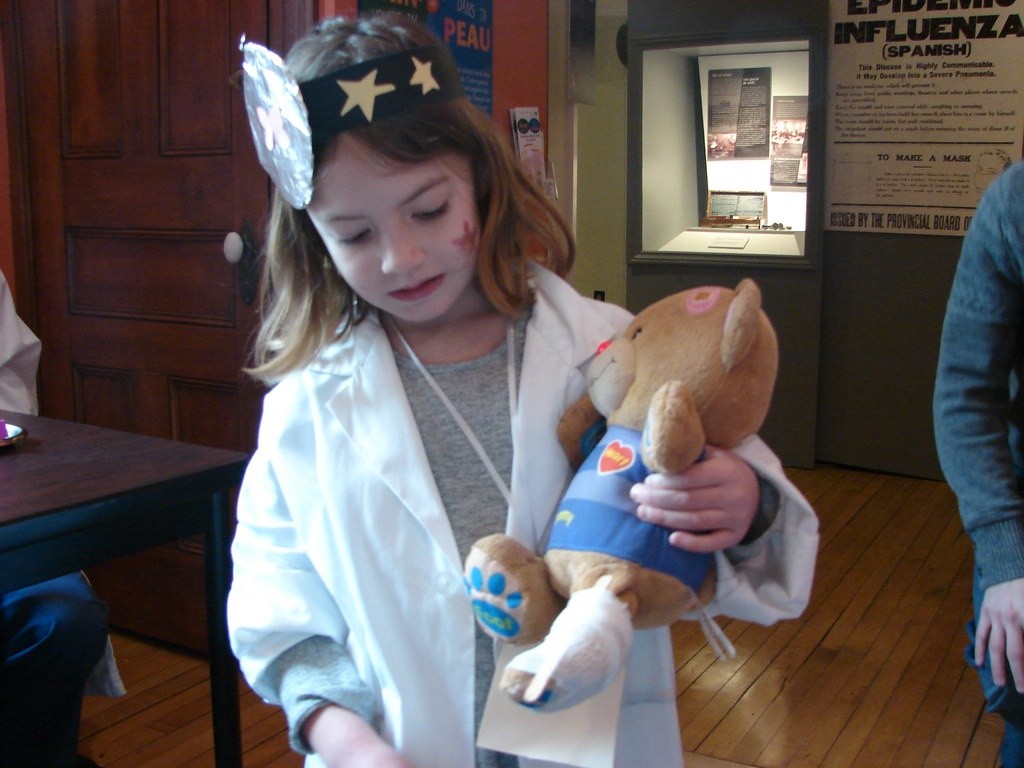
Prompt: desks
1,407,252,768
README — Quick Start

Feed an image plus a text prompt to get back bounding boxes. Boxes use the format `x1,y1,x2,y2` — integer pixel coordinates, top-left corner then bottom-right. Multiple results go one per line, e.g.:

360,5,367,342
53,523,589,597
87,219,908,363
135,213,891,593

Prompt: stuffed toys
465,279,777,713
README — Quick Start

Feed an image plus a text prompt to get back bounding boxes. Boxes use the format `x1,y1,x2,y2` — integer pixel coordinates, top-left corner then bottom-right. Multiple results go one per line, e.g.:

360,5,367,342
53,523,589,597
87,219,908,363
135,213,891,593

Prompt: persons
933,159,1024,768
0,270,126,768
226,15,818,768
